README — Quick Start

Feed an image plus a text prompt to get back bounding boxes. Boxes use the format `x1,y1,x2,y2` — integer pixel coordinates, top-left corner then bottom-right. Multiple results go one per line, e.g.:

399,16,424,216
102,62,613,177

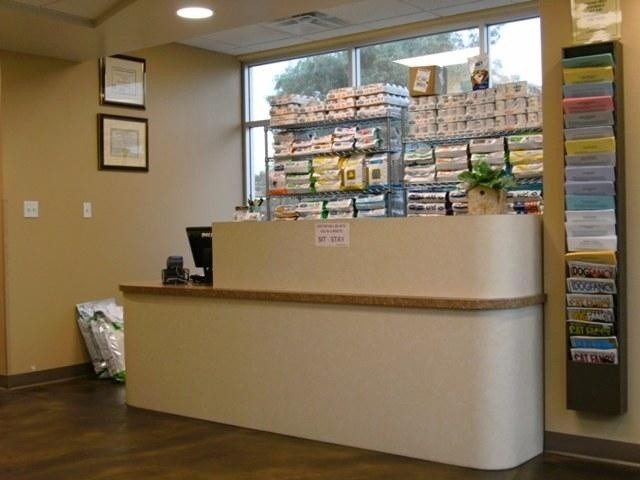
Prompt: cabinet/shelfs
401,105,543,217
263,110,401,220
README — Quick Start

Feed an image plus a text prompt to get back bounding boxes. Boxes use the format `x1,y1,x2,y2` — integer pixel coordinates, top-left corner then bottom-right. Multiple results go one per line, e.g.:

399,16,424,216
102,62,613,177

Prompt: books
562,50,619,365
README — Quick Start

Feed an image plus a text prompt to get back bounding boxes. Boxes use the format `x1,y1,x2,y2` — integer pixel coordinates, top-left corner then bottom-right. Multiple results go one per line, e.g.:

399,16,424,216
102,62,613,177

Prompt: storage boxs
408,65,447,97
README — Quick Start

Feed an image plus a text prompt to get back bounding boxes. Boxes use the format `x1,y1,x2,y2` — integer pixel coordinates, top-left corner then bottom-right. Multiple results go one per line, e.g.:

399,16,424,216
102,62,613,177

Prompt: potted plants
458,160,515,216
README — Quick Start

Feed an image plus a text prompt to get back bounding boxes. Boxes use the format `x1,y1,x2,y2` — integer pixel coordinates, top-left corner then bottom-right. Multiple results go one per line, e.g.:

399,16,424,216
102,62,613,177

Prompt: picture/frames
99,54,146,109
96,112,149,172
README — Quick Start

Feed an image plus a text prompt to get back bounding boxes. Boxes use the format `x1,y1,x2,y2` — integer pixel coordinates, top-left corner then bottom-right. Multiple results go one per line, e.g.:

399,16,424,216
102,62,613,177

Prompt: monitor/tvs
186,226,212,283
